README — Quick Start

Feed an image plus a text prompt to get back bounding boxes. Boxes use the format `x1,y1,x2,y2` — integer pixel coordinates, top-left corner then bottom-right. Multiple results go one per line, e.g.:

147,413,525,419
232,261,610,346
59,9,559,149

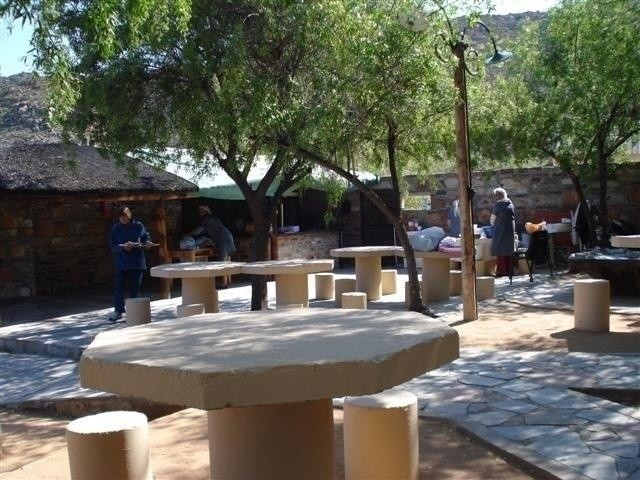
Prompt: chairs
508,231,553,285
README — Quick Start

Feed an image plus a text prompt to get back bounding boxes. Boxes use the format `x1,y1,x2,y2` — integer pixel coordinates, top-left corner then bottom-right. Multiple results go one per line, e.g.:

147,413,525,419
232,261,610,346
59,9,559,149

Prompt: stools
177,304,204,317
66,409,153,480
335,279,356,306
450,270,462,295
125,298,151,325
342,292,367,309
381,270,397,294
315,275,333,299
343,390,419,480
476,276,494,300
573,278,610,331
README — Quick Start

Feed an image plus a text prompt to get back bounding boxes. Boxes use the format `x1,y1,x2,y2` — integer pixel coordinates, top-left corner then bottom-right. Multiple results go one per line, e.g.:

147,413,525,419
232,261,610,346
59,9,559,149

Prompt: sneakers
110,313,121,320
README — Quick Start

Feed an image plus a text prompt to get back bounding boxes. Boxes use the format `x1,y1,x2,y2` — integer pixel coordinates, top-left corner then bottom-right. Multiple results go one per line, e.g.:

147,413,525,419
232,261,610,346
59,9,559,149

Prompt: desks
168,248,214,262
331,246,403,301
611,235,640,249
396,249,453,301
150,261,244,313
244,259,335,310
80,306,459,480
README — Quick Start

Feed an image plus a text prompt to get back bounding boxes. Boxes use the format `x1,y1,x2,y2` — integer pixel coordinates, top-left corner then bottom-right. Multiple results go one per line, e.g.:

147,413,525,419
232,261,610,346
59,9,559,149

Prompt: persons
188,203,238,290
107,203,156,325
445,198,461,239
473,207,495,239
490,186,517,278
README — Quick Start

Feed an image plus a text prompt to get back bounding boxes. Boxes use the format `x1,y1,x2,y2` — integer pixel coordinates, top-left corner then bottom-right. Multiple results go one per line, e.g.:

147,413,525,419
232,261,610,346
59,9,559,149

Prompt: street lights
399,0,513,321
